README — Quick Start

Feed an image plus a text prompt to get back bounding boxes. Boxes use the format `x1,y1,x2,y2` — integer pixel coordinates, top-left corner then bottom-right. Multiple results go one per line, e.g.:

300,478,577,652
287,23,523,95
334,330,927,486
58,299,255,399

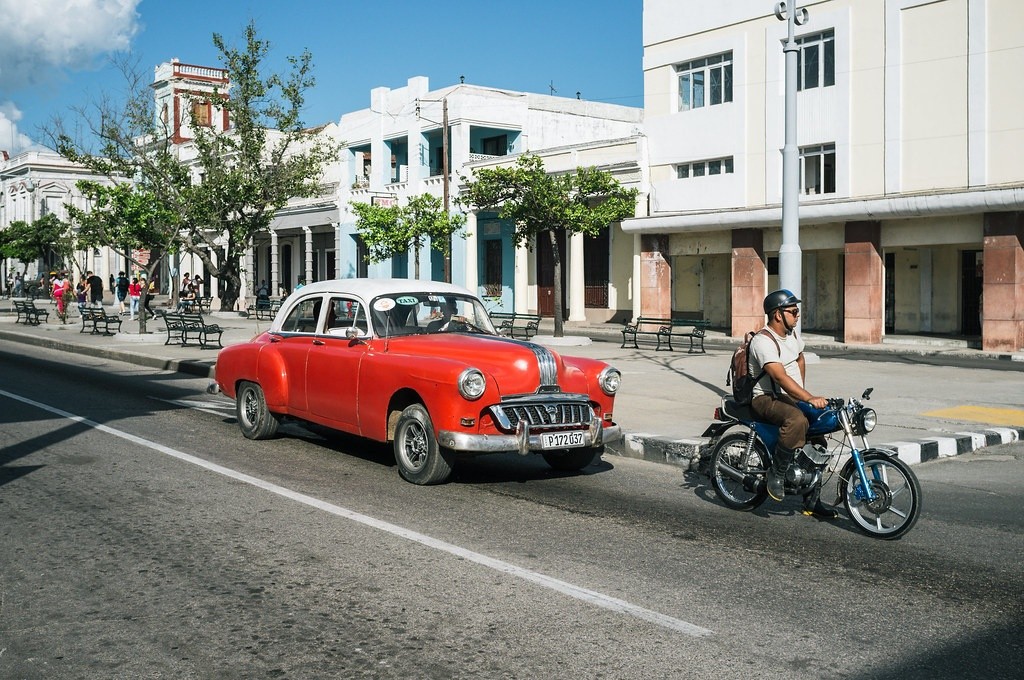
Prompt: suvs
23,271,46,295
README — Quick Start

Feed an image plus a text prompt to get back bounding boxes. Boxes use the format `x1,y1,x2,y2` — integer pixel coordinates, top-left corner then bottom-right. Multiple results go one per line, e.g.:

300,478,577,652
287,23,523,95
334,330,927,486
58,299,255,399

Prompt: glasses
780,308,800,318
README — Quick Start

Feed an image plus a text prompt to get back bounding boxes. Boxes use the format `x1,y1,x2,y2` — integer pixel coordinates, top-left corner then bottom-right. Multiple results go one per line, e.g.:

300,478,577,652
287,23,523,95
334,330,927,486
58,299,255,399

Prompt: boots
766,441,797,503
801,474,840,519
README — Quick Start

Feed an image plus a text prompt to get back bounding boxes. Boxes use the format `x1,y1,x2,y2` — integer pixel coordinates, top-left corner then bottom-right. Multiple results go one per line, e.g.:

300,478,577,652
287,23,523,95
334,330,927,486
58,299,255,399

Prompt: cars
207,277,622,485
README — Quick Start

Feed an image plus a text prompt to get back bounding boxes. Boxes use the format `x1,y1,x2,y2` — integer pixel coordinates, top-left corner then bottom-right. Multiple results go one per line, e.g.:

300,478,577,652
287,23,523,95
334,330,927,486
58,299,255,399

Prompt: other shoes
151,314,157,320
118,311,126,316
128,317,136,322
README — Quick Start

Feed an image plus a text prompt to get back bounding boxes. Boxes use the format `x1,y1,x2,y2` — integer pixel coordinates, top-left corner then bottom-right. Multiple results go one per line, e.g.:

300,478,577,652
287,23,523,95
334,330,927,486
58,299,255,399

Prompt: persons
296,280,305,291
746,291,838,518
50,270,104,322
38,273,45,295
7,272,22,298
312,302,338,335
173,272,203,316
109,271,156,321
279,283,287,301
256,280,270,301
426,299,470,333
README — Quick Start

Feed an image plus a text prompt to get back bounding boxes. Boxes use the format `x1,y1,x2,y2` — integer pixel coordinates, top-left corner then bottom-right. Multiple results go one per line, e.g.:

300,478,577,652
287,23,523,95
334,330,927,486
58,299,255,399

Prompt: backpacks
726,329,782,406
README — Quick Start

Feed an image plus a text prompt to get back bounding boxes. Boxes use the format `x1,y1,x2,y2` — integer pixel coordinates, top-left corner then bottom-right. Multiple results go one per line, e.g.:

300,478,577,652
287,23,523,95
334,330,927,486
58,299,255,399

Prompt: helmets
762,288,804,315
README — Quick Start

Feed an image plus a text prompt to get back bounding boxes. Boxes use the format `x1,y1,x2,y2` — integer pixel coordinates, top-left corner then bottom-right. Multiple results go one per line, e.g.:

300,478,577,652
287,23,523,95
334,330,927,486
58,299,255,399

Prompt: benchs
13,300,50,326
247,300,282,320
283,318,367,332
161,313,224,350
78,306,122,336
191,297,214,315
488,311,543,341
22,285,41,301
621,316,711,353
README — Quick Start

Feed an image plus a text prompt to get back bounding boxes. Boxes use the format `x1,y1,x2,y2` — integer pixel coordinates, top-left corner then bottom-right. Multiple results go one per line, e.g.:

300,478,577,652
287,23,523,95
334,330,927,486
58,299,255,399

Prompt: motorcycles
701,387,924,539
